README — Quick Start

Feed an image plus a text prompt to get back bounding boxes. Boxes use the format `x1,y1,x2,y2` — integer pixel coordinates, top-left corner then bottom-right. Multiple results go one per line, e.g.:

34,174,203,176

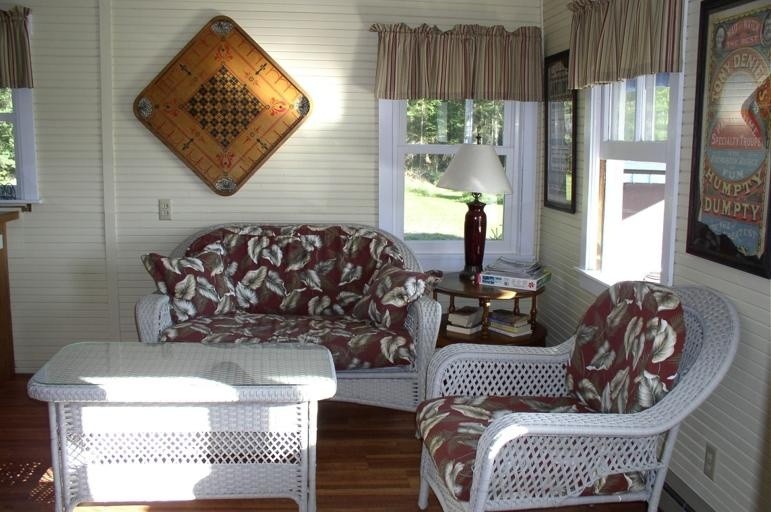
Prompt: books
478,256,552,292
446,305,484,334
487,309,533,338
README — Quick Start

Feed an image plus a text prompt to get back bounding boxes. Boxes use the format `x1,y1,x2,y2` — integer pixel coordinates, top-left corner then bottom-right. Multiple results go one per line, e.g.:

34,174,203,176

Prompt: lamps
437,144,513,284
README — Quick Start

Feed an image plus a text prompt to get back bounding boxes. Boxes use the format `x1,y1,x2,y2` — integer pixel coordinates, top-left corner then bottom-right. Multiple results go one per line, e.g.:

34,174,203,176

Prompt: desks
27,341,338,511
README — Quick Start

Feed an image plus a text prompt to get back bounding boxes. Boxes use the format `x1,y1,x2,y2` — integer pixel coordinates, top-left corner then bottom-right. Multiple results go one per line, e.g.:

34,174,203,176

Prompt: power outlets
703,441,716,480
159,199,172,221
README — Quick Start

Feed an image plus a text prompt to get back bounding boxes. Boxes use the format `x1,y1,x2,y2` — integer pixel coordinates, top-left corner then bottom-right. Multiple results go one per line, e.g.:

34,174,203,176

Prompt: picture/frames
685,0,771,279
543,48,577,213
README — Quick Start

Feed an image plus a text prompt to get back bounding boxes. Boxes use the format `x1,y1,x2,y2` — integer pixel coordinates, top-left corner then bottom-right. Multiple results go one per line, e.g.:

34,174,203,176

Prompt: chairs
134,220,443,436
417,281,742,512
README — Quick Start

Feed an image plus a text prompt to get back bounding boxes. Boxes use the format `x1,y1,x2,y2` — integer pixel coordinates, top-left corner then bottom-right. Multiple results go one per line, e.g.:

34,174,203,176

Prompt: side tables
432,270,548,347
